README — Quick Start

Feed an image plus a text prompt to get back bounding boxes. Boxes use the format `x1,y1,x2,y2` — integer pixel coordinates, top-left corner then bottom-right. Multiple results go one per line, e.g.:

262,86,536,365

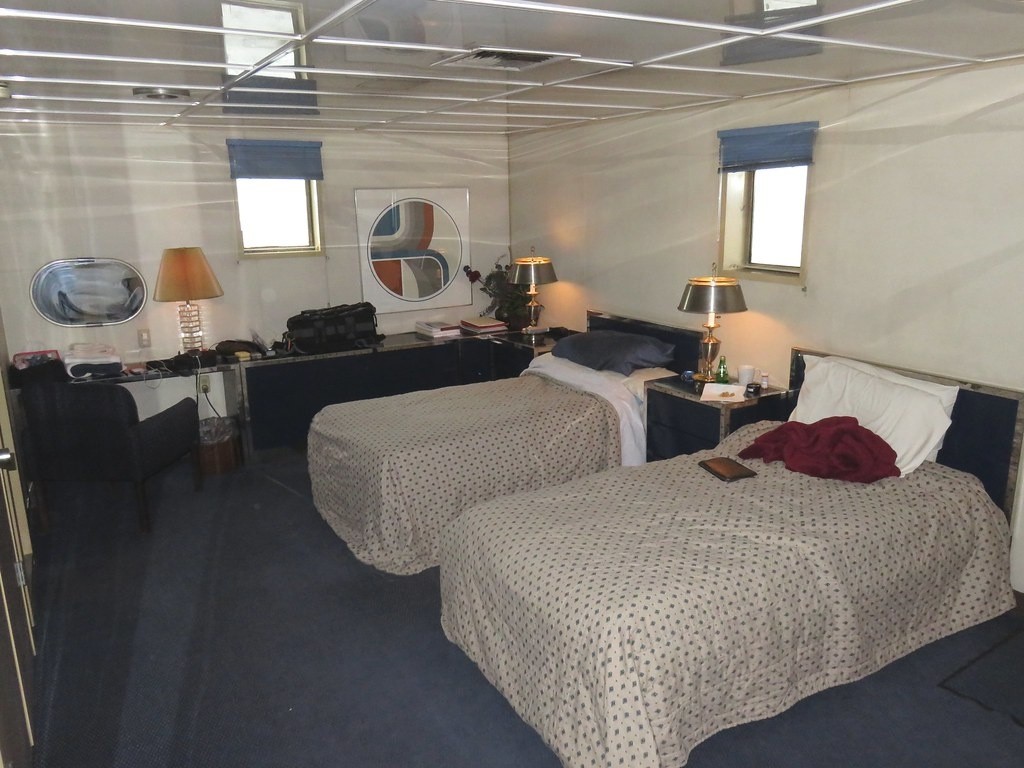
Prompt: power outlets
198,375,211,393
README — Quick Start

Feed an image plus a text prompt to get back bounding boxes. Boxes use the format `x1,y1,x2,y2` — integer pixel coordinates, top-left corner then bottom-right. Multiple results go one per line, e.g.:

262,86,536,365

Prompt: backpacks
282,302,385,355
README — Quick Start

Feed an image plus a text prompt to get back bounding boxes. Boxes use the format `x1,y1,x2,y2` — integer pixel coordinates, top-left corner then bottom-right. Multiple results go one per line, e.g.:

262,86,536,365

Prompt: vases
496,304,531,333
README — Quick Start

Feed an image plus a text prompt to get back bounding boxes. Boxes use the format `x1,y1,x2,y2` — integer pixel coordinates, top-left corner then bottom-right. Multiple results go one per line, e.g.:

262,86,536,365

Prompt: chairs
20,381,205,537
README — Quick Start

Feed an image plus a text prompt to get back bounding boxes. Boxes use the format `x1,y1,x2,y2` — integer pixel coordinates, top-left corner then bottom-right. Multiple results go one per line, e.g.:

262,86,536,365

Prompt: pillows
786,355,952,478
551,330,678,377
823,356,960,463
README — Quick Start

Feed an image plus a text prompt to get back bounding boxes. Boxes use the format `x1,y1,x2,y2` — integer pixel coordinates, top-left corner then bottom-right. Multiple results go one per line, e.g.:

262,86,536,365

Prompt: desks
238,326,505,468
10,364,248,467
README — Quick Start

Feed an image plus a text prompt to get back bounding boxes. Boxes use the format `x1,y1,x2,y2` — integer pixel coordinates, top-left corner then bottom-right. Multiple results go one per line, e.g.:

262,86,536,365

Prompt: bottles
716,356,729,384
761,373,769,390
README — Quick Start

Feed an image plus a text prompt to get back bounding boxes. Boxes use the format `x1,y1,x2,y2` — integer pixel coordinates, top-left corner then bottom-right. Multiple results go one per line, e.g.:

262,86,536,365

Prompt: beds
439,348,1024,768
307,309,708,575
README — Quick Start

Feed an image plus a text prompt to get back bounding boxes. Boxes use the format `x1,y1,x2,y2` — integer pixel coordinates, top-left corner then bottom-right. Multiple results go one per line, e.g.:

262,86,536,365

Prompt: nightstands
489,333,558,381
644,373,795,463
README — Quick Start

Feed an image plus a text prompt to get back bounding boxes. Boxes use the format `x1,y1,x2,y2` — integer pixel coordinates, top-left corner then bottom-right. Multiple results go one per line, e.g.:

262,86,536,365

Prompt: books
415,321,461,338
460,316,509,334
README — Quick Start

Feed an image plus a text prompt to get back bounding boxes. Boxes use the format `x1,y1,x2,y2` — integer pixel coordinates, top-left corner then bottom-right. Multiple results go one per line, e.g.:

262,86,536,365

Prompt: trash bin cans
195,417,243,475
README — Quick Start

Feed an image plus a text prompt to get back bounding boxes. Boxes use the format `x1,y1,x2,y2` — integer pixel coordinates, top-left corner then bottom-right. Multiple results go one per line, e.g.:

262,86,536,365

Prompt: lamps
504,247,558,334
676,276,748,382
153,247,225,354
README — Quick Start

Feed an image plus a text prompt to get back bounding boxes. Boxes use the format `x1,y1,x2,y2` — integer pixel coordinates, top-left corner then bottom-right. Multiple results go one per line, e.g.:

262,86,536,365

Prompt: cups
737,364,761,385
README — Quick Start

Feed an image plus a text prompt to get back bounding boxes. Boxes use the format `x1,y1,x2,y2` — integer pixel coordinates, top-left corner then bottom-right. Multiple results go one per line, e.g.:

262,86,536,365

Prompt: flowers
463,245,532,316
